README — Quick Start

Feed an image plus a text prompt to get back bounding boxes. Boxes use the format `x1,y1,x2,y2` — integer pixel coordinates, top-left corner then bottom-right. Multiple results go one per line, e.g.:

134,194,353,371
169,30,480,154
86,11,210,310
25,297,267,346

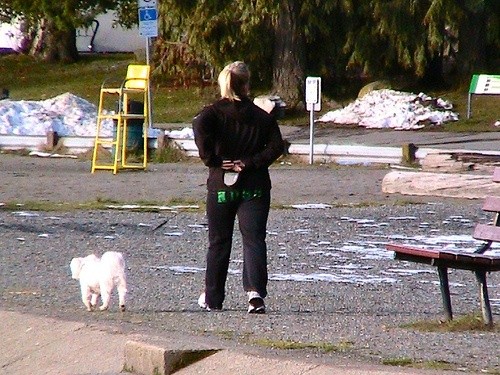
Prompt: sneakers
247,291,266,314
198,292,222,311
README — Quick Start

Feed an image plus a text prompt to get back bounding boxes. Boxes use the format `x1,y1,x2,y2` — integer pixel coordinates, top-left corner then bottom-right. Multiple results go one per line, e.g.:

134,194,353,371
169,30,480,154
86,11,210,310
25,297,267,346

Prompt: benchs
385,167,500,324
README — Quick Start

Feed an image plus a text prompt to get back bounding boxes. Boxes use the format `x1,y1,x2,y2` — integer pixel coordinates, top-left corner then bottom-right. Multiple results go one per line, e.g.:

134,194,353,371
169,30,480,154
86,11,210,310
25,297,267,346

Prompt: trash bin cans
112,99,146,160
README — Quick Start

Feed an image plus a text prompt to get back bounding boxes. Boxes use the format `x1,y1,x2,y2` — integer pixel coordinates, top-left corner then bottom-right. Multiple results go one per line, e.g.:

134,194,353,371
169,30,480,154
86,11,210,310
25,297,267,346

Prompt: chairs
91,64,151,115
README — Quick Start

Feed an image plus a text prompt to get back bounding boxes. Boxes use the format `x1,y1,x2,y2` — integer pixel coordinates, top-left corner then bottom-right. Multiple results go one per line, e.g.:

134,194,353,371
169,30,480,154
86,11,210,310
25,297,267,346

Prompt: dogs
70,251,128,312
